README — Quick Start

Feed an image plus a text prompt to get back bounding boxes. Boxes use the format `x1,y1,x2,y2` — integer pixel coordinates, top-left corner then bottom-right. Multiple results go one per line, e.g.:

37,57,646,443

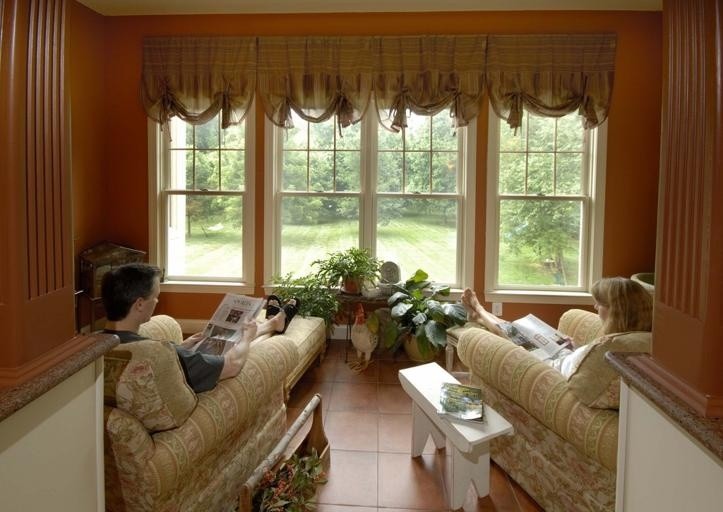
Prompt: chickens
348,302,381,373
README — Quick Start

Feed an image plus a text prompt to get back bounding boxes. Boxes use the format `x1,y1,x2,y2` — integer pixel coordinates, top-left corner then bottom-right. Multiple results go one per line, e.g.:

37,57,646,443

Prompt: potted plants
310,246,385,294
375,270,468,362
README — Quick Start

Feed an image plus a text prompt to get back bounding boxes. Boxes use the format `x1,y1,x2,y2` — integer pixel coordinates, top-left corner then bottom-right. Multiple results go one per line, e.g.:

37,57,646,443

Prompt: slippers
275,297,300,334
266,295,281,319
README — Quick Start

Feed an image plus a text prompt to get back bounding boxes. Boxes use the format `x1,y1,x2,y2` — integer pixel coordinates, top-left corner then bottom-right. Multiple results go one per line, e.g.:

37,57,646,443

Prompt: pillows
567,330,653,409
103,340,199,433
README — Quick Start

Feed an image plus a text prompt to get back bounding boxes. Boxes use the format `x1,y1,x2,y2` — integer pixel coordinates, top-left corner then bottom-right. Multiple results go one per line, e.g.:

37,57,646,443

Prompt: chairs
456,307,618,512
103,315,300,512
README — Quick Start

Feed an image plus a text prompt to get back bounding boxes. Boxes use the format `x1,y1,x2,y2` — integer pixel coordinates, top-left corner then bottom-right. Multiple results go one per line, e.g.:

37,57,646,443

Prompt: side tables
337,290,395,365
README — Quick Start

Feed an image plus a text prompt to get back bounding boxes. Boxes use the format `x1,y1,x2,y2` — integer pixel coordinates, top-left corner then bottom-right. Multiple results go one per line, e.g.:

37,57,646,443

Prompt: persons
101,261,300,392
460,275,652,369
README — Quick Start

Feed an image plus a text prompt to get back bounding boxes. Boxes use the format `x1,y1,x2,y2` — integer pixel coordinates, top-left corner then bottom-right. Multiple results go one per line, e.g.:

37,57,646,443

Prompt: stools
252,309,326,403
399,362,514,512
446,321,487,371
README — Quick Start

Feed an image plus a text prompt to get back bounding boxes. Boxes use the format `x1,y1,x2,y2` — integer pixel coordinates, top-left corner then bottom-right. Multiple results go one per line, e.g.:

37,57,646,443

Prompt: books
494,313,571,360
437,382,485,424
